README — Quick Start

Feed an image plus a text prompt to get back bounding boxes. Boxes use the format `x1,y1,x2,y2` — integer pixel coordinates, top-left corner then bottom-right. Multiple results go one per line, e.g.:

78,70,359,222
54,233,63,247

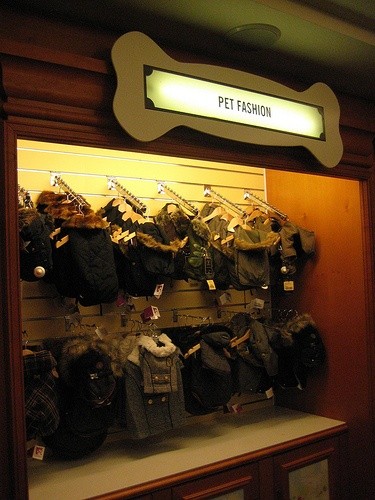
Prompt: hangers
17,189,317,379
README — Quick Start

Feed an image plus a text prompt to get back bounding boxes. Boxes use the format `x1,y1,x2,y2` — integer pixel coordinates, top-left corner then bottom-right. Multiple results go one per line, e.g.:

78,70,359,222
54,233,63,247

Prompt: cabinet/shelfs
27,403,350,500
